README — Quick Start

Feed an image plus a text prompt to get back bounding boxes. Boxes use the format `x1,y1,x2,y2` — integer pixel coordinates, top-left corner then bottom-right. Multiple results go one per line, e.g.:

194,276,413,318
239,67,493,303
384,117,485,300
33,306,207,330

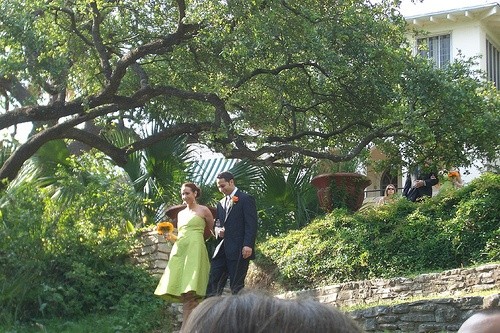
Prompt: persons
457,309,500,332
402,159,439,203
180,294,361,333
430,159,463,196
153,183,214,333
377,184,401,206
204,171,257,301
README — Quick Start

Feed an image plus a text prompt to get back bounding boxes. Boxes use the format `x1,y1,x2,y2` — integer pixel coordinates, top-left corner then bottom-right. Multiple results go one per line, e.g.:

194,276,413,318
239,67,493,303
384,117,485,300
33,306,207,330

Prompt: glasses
388,188,394,190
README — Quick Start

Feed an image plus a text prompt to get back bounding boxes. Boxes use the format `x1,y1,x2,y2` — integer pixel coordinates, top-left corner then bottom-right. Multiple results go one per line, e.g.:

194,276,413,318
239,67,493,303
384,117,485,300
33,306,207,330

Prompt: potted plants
309,150,372,212
165,177,218,242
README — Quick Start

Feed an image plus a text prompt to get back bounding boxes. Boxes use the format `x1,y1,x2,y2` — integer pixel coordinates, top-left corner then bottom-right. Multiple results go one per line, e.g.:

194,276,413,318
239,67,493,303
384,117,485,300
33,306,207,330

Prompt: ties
225,195,231,222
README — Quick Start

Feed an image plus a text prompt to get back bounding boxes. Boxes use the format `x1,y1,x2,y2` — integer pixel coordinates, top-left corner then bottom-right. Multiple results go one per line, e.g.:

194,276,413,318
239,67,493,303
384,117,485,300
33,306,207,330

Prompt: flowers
157,222,174,243
230,196,239,205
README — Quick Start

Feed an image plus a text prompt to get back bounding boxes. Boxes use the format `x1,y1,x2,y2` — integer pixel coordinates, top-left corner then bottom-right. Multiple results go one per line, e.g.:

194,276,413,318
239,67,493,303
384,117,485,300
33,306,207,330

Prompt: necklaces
184,203,199,210
